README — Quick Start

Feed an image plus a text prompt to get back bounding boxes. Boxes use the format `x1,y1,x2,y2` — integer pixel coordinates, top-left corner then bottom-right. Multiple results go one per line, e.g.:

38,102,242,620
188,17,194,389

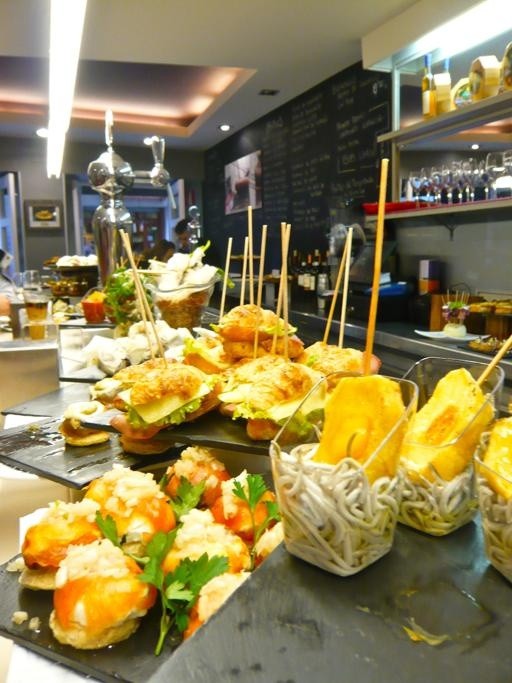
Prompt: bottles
289,244,332,298
421,52,452,125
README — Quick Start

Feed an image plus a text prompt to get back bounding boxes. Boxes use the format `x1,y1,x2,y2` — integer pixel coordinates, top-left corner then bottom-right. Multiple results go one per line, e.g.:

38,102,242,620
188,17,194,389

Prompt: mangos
311,367,512,500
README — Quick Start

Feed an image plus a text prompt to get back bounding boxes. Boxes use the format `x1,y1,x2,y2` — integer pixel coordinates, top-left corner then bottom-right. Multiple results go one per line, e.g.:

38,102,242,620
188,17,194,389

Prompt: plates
416,327,490,344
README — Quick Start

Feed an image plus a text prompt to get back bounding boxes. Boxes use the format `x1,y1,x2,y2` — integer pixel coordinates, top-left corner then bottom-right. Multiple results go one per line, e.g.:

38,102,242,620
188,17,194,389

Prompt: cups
142,271,223,331
21,289,49,321
78,300,108,325
407,147,511,212
6,293,27,341
22,268,44,289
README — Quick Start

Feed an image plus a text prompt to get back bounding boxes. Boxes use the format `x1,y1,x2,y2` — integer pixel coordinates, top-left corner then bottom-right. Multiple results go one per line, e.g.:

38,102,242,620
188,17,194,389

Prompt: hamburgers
61,304,381,455
19,442,286,649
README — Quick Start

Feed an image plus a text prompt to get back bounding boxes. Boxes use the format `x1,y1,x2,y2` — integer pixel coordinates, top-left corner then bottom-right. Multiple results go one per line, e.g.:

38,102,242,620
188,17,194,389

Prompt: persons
0,249,19,291
172,216,219,267
139,236,176,270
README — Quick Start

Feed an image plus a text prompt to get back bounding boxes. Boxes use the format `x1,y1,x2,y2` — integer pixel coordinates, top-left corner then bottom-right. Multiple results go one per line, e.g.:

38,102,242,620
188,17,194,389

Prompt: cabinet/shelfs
364,36,511,222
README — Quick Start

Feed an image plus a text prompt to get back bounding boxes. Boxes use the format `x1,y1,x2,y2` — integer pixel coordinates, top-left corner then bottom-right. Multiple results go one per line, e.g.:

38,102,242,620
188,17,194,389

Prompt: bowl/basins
45,263,98,282
